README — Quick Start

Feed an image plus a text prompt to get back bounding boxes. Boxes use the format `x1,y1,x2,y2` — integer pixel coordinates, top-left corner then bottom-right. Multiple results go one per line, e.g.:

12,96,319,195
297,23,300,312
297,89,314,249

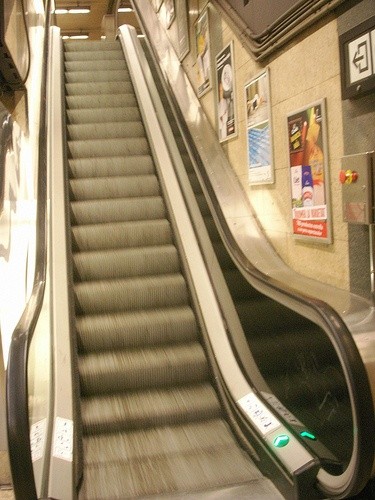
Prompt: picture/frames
151,0,166,15
283,98,335,247
192,6,214,97
159,0,176,30
241,67,278,190
174,0,192,63
213,40,240,143
